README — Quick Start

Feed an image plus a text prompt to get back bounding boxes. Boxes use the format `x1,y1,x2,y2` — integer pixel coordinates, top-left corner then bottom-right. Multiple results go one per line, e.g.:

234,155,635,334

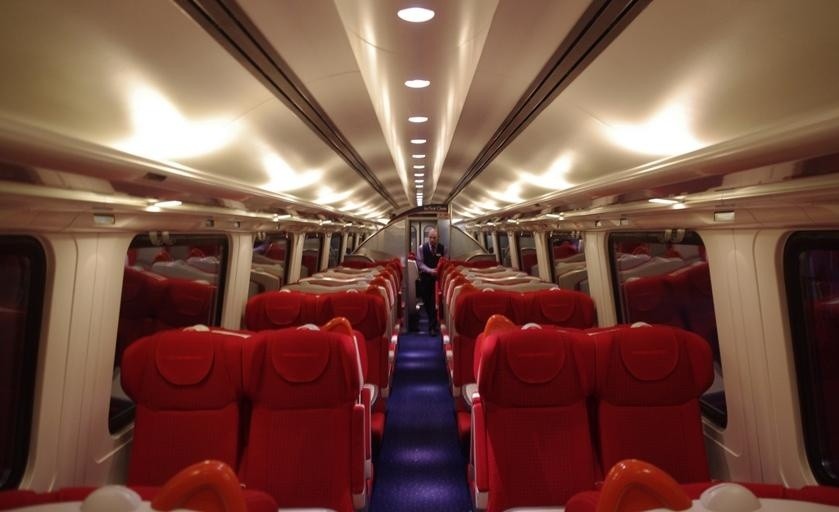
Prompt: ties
431,245,435,256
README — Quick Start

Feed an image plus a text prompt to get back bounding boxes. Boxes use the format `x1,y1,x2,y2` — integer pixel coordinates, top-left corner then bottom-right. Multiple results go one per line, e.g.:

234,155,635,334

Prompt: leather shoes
430,328,436,336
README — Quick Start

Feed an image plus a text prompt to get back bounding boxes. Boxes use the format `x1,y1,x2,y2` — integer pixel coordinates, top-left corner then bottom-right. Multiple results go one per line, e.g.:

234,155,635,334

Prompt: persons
416,228,448,336
